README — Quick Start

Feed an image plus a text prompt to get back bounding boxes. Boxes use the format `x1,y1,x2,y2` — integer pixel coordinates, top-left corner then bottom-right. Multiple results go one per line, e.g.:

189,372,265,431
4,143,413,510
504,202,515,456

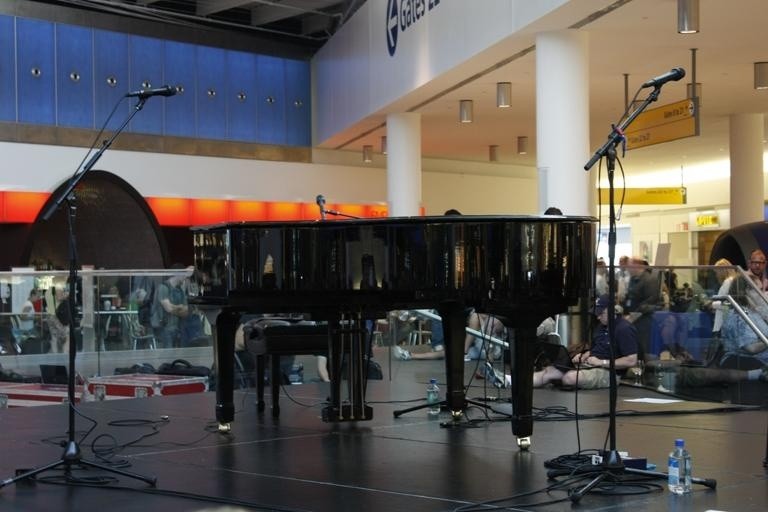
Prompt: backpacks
138,280,185,329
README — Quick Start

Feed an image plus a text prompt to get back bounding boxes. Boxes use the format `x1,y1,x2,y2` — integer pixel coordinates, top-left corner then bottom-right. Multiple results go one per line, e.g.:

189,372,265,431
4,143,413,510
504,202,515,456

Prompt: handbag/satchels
55,298,78,326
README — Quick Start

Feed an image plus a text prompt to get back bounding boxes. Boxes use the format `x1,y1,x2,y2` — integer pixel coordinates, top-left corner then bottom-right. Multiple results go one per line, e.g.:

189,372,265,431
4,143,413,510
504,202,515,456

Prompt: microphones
128,85,177,97
641,68,685,88
317,195,326,221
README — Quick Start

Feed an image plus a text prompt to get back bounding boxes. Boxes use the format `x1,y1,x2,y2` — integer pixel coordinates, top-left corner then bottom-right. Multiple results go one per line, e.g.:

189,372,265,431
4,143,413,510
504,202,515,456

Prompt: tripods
547,86,717,503
0,98,158,487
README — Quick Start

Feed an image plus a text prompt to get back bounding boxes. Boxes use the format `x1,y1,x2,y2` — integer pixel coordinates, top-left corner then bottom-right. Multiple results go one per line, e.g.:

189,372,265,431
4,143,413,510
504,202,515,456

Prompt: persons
314,313,383,382
255,312,302,391
396,208,566,359
478,248,768,391
0,263,212,355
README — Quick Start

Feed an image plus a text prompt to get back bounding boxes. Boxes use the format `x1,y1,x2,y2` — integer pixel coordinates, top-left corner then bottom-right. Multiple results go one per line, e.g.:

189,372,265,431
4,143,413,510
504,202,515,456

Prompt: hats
586,293,616,318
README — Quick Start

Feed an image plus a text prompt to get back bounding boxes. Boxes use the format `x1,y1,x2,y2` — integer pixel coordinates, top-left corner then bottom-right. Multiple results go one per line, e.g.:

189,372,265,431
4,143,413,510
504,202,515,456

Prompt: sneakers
392,345,412,362
481,361,509,389
464,353,471,362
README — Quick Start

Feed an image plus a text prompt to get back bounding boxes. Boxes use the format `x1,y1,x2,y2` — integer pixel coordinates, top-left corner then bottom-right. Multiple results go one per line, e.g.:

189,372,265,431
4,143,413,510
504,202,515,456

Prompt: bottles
427,379,440,417
667,439,693,495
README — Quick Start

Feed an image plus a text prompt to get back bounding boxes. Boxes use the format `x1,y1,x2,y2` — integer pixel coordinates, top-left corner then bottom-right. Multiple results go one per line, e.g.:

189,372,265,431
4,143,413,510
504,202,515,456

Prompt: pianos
184,215,598,448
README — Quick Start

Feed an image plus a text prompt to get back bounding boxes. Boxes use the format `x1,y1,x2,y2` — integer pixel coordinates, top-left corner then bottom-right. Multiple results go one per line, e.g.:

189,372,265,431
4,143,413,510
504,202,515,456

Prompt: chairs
9,307,159,353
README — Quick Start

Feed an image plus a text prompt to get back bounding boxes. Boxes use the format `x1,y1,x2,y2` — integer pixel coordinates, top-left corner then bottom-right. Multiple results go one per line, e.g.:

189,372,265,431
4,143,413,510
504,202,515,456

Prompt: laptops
544,343,598,369
